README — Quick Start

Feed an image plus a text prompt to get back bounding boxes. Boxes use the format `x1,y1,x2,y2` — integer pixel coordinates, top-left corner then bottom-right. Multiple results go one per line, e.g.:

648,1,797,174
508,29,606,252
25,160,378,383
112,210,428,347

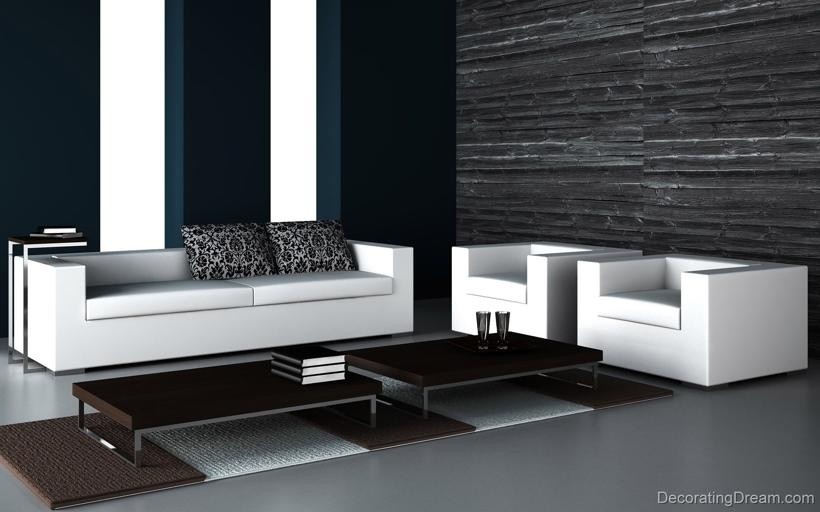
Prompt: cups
476,310,491,350
495,310,511,350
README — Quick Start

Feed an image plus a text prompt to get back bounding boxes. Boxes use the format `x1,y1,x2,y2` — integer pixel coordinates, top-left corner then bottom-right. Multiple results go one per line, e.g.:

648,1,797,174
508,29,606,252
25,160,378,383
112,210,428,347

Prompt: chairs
445,238,810,392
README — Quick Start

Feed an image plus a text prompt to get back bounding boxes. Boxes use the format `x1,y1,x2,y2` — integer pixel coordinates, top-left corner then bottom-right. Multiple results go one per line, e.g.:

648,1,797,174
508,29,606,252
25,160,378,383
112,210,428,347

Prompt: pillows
176,219,359,279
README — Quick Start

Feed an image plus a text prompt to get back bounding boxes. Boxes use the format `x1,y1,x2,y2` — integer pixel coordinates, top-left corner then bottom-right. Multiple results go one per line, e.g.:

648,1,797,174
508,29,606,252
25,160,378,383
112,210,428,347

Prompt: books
29,231,84,238
38,225,77,234
270,369,346,385
270,345,346,368
270,359,346,376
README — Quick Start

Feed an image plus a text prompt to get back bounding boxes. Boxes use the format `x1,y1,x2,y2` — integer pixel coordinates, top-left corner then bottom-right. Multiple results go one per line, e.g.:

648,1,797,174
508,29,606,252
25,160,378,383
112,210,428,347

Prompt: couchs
6,239,420,376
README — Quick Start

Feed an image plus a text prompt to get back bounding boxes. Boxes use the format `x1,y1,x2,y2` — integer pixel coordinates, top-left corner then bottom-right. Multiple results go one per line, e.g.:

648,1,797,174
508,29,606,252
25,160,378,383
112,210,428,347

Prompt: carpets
1,369,674,511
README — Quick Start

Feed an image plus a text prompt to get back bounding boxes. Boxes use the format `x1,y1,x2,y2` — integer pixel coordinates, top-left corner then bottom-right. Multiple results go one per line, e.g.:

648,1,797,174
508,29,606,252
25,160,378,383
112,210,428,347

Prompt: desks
72,329,605,470
2,232,91,375
